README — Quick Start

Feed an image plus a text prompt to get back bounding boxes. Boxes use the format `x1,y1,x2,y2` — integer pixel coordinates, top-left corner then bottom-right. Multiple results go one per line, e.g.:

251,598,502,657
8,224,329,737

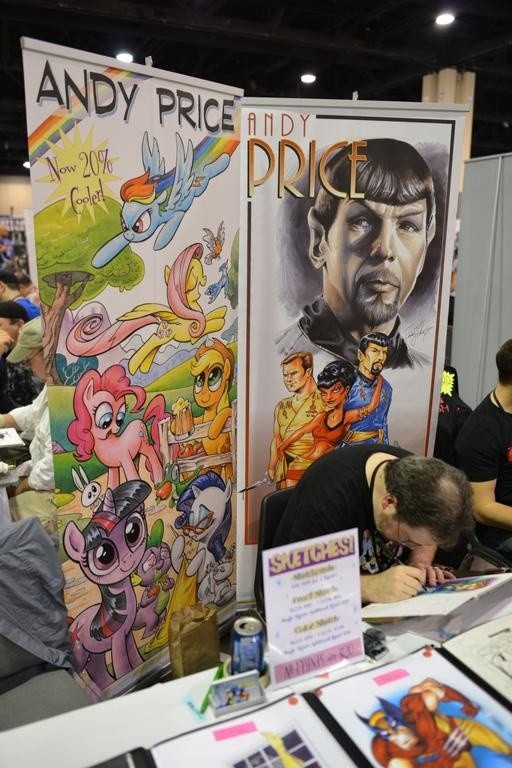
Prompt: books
361,567,512,617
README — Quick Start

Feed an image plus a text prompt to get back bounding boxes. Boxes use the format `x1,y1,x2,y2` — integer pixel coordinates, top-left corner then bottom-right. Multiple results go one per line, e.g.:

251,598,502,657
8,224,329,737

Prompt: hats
0,301,26,319
6,317,44,366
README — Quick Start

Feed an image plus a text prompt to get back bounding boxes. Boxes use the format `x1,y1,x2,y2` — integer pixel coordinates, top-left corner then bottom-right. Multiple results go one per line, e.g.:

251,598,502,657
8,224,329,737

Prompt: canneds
230,616,264,676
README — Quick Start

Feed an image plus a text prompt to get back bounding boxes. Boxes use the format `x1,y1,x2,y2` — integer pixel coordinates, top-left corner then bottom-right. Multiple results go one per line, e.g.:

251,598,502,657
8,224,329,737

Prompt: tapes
222,656,271,689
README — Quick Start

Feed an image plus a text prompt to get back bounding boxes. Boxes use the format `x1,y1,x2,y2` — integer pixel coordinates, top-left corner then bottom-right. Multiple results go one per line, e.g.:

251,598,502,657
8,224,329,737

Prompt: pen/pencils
391,556,428,593
200,662,223,714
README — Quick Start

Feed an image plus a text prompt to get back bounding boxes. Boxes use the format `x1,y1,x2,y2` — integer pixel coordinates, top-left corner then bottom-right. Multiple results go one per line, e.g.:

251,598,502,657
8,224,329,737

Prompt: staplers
361,619,390,660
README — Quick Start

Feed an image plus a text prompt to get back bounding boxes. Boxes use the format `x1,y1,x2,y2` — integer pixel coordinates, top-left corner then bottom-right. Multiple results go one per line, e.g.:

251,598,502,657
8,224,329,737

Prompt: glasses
19,348,44,369
396,515,422,549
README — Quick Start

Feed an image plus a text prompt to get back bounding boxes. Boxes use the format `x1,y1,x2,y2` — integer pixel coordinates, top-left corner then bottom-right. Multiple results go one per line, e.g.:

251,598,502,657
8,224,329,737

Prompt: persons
341,329,397,448
451,335,511,563
277,131,439,369
263,349,326,492
274,357,384,492
253,439,477,624
0,269,63,550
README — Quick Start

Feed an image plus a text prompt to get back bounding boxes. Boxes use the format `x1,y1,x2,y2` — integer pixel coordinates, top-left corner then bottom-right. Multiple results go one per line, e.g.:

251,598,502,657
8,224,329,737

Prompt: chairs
246,485,296,636
